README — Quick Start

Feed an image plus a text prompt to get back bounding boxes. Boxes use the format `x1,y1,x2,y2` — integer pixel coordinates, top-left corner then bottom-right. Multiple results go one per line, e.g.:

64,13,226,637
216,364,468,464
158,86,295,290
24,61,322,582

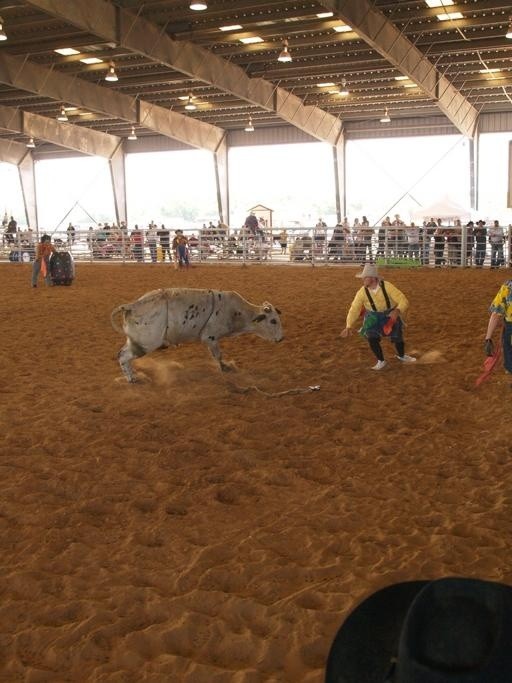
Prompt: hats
355,264,384,280
322,576,511,681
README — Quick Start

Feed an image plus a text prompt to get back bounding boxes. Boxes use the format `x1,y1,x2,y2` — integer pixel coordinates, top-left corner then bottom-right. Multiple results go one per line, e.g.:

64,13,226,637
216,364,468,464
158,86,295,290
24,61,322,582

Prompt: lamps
58,105,68,121
380,106,391,122
104,60,119,81
185,91,197,110
277,37,292,61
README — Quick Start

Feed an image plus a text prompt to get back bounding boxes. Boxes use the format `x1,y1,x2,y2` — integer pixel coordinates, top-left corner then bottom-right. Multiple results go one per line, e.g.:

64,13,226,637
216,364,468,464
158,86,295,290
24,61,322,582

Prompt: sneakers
371,360,388,370
397,354,416,362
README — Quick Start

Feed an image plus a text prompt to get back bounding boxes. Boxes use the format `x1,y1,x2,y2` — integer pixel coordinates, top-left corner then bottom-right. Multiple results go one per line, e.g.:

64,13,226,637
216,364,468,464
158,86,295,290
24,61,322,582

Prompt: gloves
485,338,496,357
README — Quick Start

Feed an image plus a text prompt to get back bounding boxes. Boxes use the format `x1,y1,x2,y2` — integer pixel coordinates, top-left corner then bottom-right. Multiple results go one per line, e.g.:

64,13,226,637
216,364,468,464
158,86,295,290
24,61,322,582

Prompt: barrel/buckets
50,251,74,285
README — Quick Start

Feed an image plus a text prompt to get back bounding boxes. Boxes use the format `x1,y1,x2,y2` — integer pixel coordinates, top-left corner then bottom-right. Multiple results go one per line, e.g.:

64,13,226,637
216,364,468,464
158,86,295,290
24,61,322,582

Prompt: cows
110,286,284,384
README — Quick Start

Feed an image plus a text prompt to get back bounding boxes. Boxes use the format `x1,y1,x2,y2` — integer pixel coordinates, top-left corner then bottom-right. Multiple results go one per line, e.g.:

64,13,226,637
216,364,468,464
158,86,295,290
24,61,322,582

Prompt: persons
30,233,57,286
1,210,512,269
483,276,512,378
339,263,417,369
